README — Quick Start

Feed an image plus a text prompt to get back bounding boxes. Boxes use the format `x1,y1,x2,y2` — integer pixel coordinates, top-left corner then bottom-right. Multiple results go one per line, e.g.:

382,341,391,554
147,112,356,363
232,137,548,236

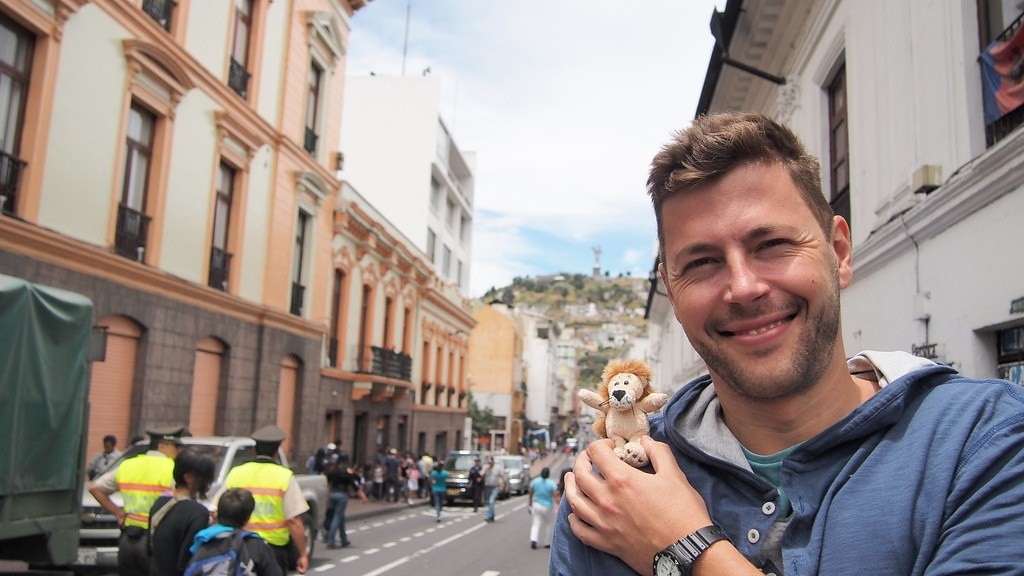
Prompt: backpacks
183,530,260,576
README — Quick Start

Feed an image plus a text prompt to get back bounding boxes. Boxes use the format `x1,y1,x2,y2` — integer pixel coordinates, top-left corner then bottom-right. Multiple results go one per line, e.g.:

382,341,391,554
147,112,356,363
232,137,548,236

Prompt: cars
442,451,509,506
492,456,532,498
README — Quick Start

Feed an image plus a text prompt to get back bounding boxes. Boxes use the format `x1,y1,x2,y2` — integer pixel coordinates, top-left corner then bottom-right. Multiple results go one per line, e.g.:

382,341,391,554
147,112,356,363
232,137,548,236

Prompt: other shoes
544,545,551,549
484,515,495,522
474,505,478,512
327,541,350,549
434,514,442,522
531,541,536,549
408,499,413,505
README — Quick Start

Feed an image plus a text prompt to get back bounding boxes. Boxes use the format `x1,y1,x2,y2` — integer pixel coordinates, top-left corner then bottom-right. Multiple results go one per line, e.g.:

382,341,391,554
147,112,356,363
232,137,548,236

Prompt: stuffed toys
579,357,668,467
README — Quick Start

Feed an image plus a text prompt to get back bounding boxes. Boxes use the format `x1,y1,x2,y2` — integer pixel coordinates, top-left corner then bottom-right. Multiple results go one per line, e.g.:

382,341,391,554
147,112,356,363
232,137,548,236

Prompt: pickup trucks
79,436,330,568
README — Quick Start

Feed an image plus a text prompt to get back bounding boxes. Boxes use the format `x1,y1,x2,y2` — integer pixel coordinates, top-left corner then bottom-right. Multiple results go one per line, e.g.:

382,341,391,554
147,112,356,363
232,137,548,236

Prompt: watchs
652,525,729,576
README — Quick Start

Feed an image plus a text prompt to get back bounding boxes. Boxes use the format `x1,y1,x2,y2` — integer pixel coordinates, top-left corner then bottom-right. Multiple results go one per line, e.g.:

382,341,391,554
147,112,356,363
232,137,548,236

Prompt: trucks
0,275,96,576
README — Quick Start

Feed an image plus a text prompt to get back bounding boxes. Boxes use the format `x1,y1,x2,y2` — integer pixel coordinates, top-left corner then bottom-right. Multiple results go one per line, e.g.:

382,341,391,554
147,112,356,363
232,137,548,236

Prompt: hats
327,443,337,450
251,425,286,444
390,448,398,454
145,426,186,447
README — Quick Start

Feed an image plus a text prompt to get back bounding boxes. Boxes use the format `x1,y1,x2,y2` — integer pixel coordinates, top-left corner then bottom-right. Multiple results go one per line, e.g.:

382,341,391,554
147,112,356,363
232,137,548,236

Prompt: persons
558,468,573,499
131,434,143,444
306,438,439,549
88,426,187,576
522,420,578,464
429,463,449,521
468,458,485,512
528,467,561,549
183,488,284,576
206,424,309,576
477,455,505,522
549,110,1024,576
147,451,215,576
90,434,122,479
